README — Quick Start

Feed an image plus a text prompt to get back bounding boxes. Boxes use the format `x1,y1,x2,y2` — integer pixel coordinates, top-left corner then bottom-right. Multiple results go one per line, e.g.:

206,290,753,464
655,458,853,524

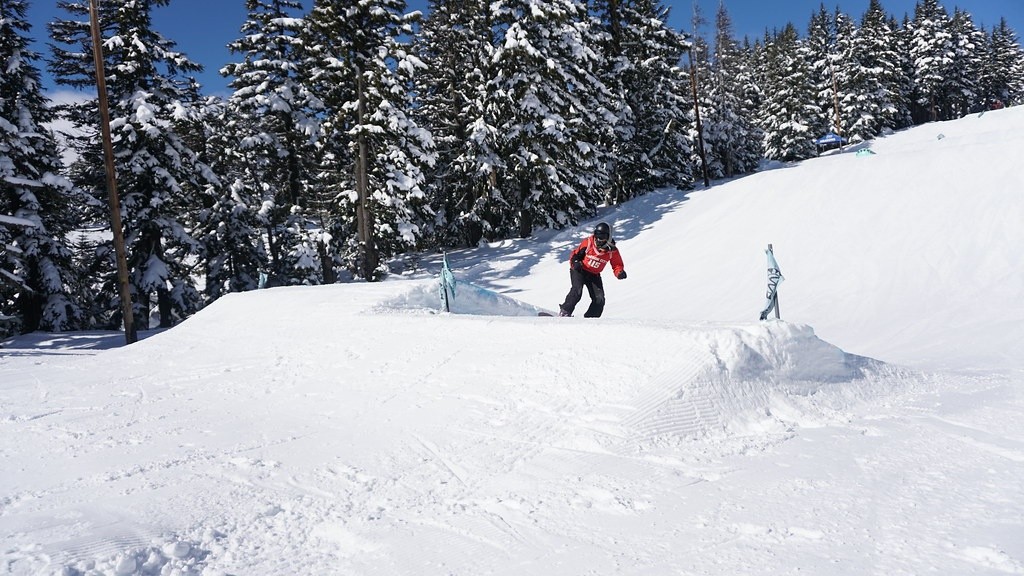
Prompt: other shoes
560,310,569,316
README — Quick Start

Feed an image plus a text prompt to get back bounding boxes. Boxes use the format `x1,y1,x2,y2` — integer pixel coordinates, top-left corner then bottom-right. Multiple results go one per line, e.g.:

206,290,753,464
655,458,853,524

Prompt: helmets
594,223,609,234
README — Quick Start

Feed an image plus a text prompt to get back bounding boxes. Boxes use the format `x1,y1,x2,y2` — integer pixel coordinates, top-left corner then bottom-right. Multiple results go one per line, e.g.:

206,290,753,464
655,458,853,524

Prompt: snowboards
538,312,553,317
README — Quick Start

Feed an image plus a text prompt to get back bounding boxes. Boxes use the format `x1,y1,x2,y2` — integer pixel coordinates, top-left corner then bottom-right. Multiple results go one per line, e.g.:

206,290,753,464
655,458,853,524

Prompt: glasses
594,233,608,240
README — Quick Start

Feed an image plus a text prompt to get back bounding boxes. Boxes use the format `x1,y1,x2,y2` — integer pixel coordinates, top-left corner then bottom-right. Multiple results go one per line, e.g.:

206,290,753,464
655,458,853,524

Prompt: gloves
573,260,583,271
618,270,627,279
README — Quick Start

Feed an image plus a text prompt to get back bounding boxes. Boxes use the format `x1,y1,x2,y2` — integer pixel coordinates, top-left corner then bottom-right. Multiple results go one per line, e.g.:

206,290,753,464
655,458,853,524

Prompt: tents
815,131,847,157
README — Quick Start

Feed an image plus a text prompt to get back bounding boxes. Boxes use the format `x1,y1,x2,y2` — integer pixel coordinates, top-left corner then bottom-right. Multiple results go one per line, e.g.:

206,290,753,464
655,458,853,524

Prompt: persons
559,221,627,318
